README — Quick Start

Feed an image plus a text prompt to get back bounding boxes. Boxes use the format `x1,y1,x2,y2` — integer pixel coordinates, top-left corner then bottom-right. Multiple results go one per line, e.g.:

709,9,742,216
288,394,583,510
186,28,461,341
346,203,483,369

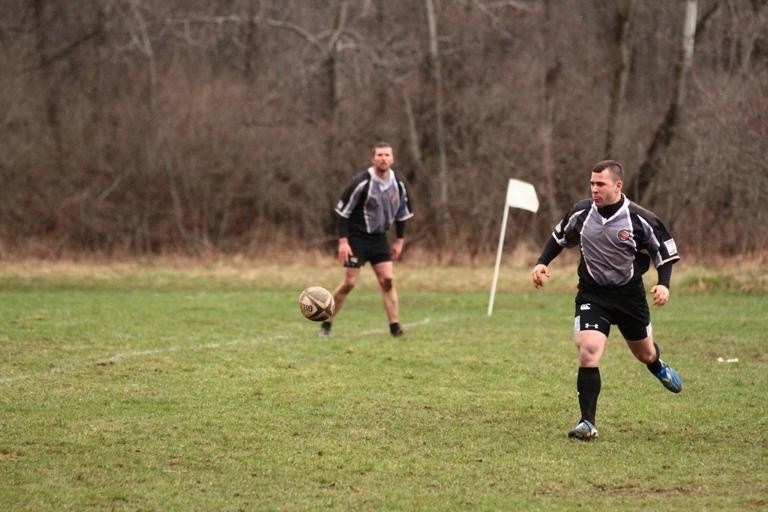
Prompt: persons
315,142,415,339
529,158,686,442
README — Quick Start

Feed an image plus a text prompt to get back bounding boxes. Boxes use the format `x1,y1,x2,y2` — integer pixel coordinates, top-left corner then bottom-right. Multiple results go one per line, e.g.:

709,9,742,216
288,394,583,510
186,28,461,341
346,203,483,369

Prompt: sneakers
568,419,599,440
391,328,404,337
318,327,331,338
647,358,682,394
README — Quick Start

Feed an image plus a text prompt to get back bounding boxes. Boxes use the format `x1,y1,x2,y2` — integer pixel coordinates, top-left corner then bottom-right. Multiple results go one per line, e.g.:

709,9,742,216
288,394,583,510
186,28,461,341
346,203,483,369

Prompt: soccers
299,286,335,321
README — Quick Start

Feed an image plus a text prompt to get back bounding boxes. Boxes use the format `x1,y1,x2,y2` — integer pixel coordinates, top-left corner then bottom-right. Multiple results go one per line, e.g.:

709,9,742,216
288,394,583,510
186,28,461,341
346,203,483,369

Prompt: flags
507,178,540,213
